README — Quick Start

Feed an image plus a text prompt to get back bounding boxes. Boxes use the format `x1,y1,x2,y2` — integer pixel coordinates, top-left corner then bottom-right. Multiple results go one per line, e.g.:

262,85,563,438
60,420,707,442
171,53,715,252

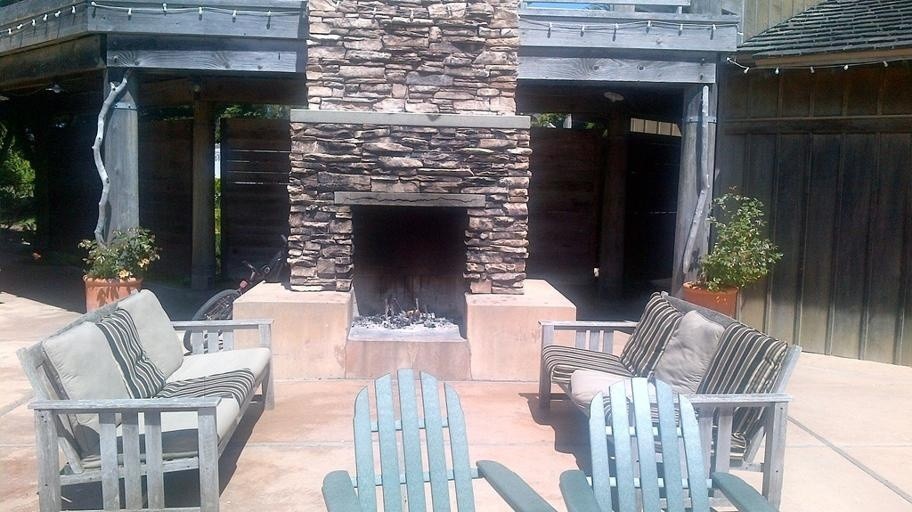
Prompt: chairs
321,368,556,512
559,377,776,511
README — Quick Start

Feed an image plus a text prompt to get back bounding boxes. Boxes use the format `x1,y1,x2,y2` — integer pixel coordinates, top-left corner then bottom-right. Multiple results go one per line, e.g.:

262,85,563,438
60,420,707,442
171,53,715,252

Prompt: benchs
536,294,802,512
15,289,277,511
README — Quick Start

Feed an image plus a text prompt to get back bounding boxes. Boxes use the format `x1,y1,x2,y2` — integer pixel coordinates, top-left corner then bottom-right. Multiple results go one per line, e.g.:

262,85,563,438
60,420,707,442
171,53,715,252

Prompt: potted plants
682,185,785,322
75,226,165,313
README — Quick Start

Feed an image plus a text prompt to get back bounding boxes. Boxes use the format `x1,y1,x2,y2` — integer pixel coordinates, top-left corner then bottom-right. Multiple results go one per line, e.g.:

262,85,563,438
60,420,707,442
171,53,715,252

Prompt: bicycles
185,234,289,352
